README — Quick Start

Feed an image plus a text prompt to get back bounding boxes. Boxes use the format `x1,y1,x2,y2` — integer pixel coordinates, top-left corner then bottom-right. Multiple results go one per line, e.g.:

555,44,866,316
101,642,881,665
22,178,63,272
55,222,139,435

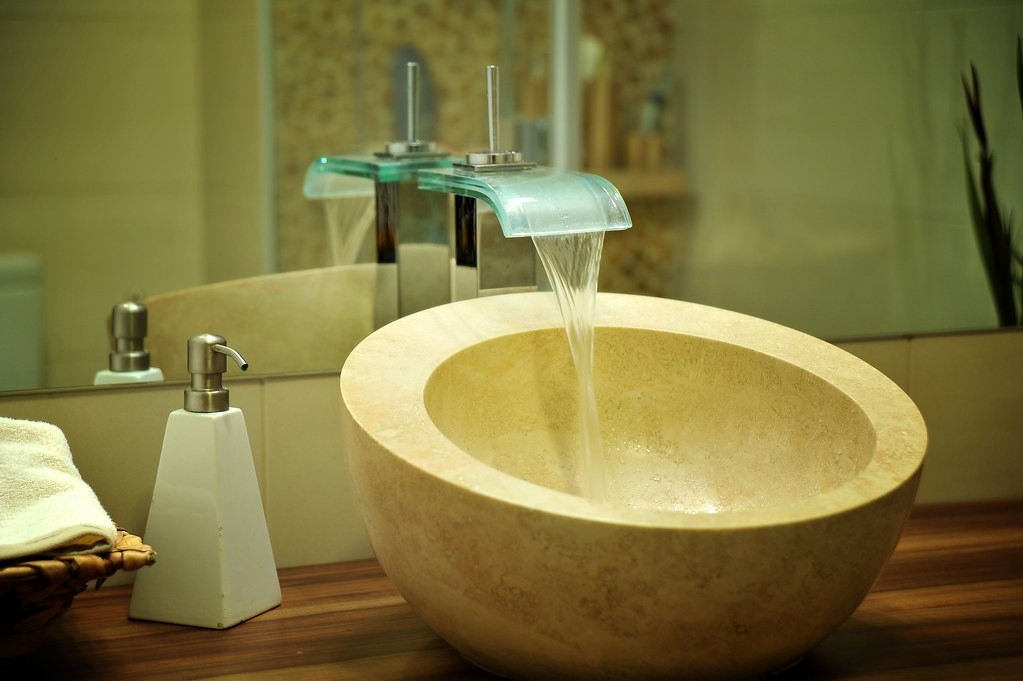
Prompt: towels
0,416,118,561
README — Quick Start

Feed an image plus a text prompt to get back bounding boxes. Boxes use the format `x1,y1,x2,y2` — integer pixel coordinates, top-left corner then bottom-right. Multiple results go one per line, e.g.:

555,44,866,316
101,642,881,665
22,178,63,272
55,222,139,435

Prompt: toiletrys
94,292,165,388
128,331,284,631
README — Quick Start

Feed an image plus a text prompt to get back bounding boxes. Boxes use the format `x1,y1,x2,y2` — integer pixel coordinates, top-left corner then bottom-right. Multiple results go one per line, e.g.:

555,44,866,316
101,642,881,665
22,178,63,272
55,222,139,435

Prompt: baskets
0,526,157,658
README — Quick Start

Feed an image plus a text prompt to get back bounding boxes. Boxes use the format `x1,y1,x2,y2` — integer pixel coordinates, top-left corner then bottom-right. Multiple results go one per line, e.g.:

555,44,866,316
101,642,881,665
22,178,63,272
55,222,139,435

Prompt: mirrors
0,0,1023,396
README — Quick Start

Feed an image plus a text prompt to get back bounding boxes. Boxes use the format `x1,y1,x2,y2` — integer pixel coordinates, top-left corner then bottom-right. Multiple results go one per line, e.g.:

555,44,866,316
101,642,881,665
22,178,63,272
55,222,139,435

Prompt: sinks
98,263,477,386
338,291,929,681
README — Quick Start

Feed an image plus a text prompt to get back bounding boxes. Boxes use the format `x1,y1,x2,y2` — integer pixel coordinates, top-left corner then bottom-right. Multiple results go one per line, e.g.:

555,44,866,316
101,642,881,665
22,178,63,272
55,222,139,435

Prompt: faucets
303,62,450,329
418,64,632,301
